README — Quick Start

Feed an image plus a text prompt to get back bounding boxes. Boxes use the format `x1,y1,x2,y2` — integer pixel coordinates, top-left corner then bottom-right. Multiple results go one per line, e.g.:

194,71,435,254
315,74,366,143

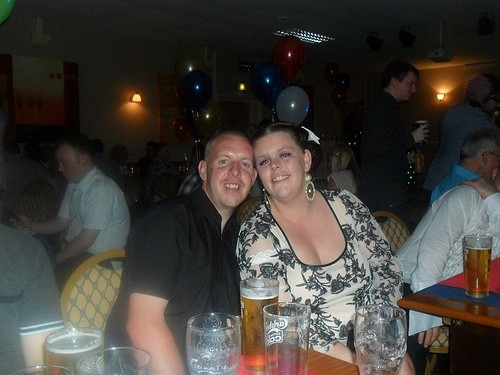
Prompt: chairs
60,247,126,332
372,211,410,253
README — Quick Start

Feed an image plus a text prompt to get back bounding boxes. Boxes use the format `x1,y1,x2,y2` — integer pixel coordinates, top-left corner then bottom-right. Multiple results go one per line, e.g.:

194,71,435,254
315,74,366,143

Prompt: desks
397,256,500,375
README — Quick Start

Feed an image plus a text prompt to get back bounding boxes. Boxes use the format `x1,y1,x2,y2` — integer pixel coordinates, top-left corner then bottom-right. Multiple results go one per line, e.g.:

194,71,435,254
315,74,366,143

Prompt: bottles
406,142,425,194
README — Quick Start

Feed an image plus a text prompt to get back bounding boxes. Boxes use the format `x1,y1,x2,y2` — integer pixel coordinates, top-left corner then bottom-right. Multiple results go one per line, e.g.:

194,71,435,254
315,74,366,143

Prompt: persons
0,135,205,249
9,137,130,283
0,223,62,375
327,90,500,205
391,166,500,375
104,130,257,375
235,122,415,375
362,60,430,223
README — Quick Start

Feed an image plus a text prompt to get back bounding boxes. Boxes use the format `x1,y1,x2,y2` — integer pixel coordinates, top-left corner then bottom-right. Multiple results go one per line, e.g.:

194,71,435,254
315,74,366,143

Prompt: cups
239,277,280,374
412,119,428,142
261,301,311,375
187,312,241,375
75,346,153,375
462,234,494,299
45,326,104,375
355,304,407,375
11,364,73,375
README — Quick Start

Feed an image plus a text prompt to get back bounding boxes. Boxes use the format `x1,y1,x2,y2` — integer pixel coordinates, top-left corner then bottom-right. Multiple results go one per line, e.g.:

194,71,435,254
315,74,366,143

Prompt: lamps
398,26,416,49
478,13,494,35
366,32,383,52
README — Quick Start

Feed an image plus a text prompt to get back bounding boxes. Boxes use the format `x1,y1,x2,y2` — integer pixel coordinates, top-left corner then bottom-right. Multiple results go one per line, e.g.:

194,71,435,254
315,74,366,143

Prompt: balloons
176,52,212,79
173,119,188,142
179,71,213,110
276,86,309,126
251,60,288,110
192,105,221,138
273,36,306,80
